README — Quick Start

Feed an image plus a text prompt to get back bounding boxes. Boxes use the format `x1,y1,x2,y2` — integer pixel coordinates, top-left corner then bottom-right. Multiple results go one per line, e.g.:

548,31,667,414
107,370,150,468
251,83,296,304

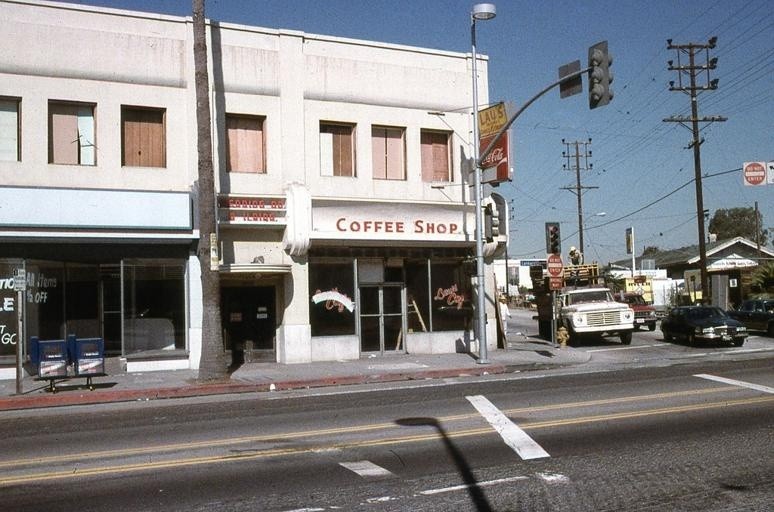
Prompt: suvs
559,286,636,347
726,298,774,337
612,290,660,332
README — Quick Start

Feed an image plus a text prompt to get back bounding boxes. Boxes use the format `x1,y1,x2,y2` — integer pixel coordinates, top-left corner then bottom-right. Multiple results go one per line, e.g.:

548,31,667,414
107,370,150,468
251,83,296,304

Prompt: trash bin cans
32,335,105,394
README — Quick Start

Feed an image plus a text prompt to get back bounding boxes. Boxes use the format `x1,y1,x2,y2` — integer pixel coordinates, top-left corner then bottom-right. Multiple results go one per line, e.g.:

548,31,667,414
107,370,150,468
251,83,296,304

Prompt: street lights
579,212,608,264
468,2,499,368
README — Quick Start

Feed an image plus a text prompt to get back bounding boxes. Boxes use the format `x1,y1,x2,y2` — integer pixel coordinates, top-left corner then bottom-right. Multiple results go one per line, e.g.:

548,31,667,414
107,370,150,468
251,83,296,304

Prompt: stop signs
546,254,565,278
742,161,767,186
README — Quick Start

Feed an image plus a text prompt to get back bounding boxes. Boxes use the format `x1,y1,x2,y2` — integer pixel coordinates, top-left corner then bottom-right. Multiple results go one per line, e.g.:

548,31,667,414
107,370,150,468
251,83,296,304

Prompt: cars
659,304,750,348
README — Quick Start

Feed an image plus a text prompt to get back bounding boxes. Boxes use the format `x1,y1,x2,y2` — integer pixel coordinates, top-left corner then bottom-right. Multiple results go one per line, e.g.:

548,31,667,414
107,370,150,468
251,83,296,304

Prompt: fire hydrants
556,324,571,349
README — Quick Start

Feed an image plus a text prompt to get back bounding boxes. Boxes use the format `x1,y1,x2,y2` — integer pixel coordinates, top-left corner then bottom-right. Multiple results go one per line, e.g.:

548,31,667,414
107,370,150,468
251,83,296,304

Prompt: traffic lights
483,202,499,244
544,221,562,255
587,39,615,111
